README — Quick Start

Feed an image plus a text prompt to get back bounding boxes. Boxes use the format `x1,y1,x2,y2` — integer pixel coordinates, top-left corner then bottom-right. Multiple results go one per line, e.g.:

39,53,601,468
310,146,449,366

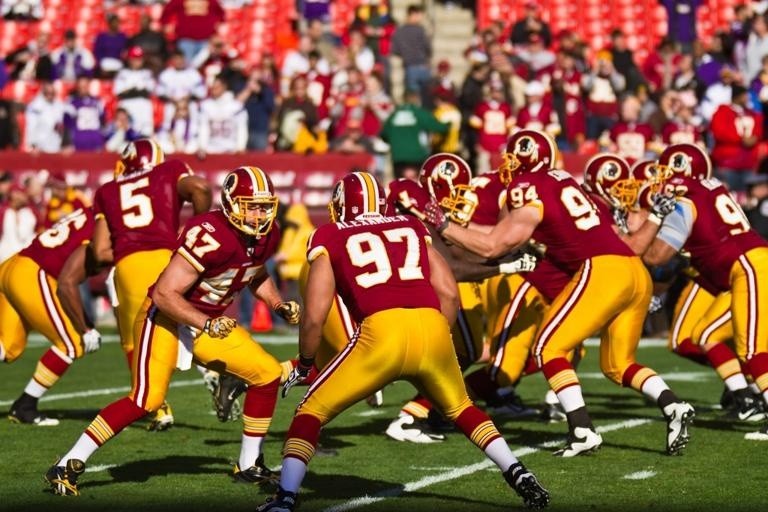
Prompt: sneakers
366,390,383,408
255,484,298,512
385,386,567,445
501,461,550,512
7,402,59,428
43,455,84,496
552,425,602,459
715,387,768,441
662,400,695,457
232,454,280,487
146,370,248,432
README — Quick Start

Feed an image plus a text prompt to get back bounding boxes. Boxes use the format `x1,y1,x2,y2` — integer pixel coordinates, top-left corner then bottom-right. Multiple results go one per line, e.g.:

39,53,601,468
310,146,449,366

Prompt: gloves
82,328,101,354
422,196,448,234
650,192,676,218
202,315,236,340
276,301,301,325
498,252,537,274
281,365,312,398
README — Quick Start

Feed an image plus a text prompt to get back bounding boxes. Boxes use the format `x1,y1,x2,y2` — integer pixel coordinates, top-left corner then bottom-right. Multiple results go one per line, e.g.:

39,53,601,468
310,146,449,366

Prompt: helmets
327,171,385,224
220,166,279,240
583,152,638,211
631,159,656,187
120,138,164,177
416,152,477,225
498,129,555,183
658,143,712,197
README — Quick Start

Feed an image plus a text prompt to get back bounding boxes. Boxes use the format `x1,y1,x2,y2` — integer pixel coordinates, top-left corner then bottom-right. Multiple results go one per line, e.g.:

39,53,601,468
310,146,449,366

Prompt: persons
0,1,768,511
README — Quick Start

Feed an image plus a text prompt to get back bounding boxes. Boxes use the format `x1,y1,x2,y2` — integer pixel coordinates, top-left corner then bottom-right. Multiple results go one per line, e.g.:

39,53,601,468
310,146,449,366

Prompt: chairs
477,0,768,68
0,0,366,153
